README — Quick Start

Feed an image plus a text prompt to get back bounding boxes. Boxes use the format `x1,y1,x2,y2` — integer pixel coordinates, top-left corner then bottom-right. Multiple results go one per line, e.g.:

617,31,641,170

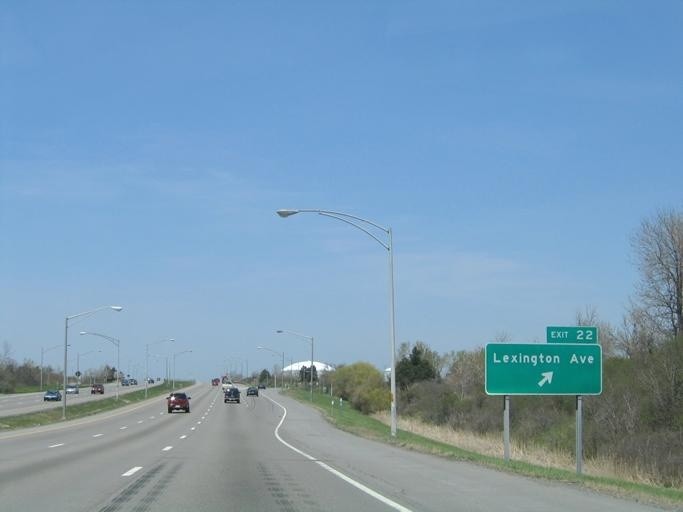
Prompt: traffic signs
485,343,604,396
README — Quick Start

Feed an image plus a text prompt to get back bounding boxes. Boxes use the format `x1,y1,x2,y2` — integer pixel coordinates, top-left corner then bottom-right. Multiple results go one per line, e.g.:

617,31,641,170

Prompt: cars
258,384,266,390
66,384,79,394
212,376,241,403
247,387,258,397
44,390,61,402
121,379,137,386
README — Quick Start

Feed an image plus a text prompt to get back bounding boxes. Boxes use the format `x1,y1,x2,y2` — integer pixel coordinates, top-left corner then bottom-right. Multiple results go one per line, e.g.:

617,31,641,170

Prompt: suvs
91,384,104,394
167,392,191,413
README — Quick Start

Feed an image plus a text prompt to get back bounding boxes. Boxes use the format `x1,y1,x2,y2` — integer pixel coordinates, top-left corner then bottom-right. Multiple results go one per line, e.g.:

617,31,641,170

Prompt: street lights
40,344,70,390
277,330,313,402
257,347,284,390
62,306,123,419
277,208,398,439
145,339,192,399
